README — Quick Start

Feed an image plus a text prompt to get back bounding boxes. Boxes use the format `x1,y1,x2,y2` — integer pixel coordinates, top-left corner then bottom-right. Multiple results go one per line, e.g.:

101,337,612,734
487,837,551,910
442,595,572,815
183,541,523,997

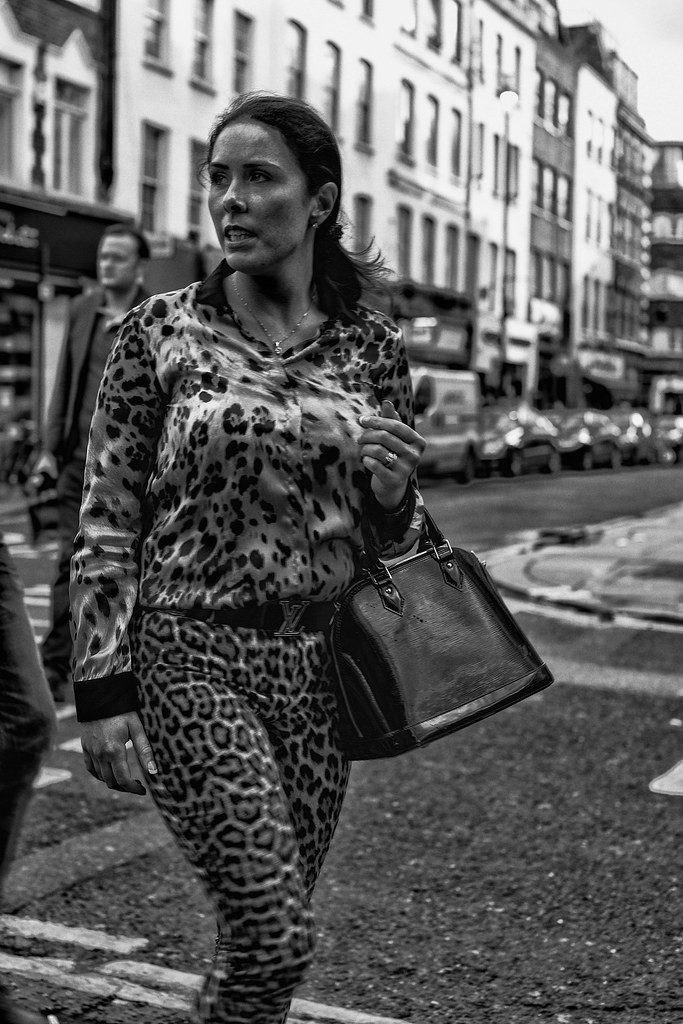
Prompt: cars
477,398,683,481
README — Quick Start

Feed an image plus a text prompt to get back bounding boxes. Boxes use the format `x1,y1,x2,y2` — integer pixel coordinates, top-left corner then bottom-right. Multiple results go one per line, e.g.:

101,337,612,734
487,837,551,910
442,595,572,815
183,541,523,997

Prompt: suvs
408,369,495,480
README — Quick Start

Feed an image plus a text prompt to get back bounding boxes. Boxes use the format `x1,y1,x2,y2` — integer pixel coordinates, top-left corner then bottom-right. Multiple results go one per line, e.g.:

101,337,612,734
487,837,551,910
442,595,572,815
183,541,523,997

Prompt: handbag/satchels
330,547,554,761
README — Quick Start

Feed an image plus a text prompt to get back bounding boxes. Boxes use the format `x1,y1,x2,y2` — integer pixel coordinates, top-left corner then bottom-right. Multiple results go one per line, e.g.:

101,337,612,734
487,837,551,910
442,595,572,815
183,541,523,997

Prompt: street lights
493,86,522,401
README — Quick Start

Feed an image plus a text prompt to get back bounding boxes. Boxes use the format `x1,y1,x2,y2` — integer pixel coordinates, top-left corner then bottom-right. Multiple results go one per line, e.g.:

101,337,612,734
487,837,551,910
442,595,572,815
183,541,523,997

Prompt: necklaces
232,273,313,355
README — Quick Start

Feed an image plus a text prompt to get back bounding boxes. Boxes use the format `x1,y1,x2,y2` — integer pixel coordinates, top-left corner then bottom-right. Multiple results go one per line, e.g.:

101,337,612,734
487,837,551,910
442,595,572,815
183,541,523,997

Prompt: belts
179,598,339,638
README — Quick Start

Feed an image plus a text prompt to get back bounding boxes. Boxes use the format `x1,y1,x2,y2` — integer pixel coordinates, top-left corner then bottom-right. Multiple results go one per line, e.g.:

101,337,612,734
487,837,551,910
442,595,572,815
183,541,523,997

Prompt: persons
67,97,427,1024
37,224,156,700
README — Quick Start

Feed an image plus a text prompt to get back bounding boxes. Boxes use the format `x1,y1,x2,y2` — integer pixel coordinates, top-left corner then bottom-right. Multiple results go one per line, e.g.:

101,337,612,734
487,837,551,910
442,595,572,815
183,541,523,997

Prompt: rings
381,451,397,468
